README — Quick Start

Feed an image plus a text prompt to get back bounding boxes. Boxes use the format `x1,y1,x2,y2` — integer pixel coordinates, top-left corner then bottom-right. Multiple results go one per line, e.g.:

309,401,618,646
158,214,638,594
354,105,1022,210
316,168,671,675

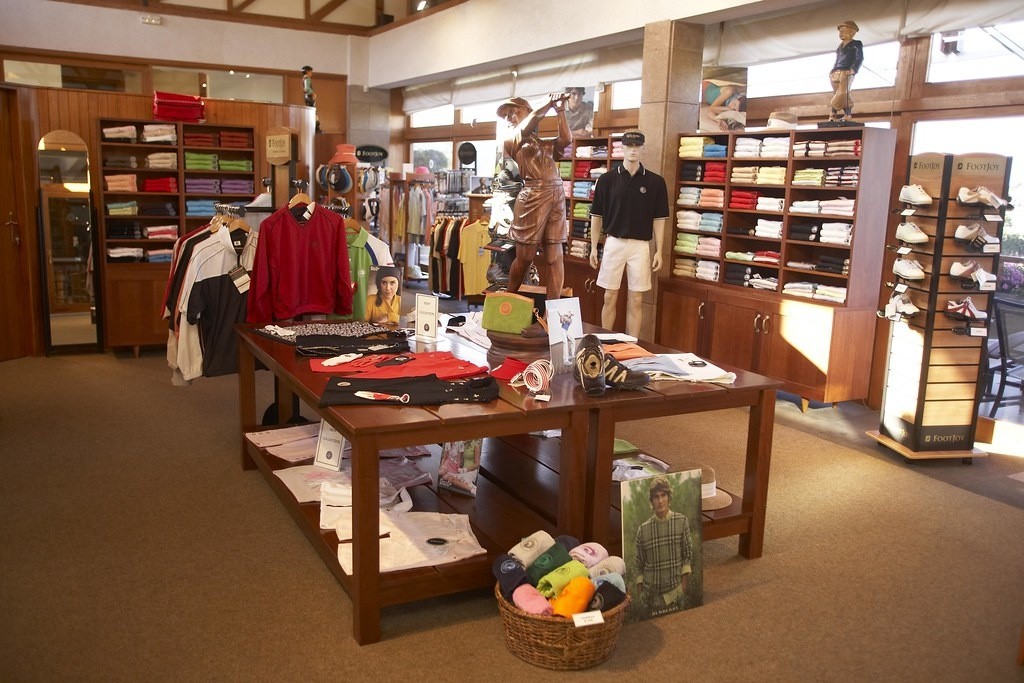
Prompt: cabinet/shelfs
14,83,316,359
865,151,1013,465
327,163,386,237
541,137,630,330
653,126,897,413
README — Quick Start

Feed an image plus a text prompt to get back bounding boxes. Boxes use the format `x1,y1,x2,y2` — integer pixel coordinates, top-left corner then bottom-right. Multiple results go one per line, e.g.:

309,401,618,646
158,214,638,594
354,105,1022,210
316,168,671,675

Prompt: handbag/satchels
481,291,549,337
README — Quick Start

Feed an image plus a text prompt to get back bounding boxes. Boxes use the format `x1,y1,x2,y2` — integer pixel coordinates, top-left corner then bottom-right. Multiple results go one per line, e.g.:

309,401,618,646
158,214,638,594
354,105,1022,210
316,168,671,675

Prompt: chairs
985,296,1024,420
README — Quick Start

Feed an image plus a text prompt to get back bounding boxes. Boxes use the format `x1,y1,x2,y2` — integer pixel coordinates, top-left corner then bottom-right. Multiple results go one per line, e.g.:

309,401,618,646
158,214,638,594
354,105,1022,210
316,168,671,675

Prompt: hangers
209,203,250,249
413,180,437,192
287,181,313,225
326,205,361,232
430,212,490,227
264,180,273,193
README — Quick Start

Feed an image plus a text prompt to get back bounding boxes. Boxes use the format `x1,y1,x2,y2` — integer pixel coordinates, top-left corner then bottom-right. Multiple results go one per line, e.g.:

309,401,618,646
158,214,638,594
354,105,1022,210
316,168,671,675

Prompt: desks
231,321,785,646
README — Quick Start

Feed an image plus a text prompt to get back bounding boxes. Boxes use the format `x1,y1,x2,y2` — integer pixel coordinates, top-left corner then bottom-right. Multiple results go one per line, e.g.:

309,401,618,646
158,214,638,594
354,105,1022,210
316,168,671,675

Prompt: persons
471,178,493,193
301,65,320,133
560,87,594,133
587,131,670,347
702,80,747,133
496,92,575,335
557,309,576,366
364,266,401,325
828,20,864,122
629,476,693,612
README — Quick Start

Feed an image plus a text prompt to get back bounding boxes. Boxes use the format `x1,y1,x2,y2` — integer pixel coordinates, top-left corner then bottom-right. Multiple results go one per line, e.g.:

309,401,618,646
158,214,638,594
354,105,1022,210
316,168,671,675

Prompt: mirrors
38,129,104,355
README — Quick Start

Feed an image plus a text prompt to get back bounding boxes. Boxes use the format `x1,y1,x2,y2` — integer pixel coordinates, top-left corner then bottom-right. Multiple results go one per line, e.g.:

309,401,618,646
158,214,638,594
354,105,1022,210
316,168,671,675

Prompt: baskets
495,579,632,670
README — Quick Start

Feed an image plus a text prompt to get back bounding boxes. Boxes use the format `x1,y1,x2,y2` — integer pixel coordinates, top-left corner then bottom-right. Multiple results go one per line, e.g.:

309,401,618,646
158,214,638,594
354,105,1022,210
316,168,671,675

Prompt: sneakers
895,222,929,244
949,261,997,281
899,184,933,205
576,335,606,397
958,186,1008,208
945,297,987,320
574,353,650,390
955,224,1000,244
889,291,920,315
892,258,925,280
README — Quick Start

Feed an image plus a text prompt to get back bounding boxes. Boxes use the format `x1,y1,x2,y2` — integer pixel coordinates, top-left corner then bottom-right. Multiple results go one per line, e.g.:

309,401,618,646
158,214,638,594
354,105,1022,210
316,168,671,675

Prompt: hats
621,132,645,145
316,163,379,222
375,267,401,286
